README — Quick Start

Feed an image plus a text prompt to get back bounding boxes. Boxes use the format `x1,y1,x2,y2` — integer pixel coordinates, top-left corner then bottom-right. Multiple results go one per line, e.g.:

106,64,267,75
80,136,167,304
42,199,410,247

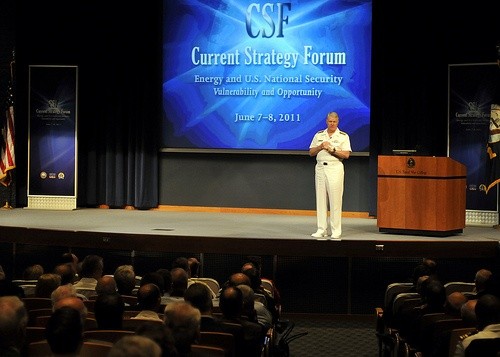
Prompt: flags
486,97,500,180
0,51,16,179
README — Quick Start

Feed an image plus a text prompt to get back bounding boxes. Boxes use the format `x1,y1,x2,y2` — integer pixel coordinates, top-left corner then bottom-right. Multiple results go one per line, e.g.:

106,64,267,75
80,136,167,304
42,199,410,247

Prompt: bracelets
377,311,383,315
331,148,337,155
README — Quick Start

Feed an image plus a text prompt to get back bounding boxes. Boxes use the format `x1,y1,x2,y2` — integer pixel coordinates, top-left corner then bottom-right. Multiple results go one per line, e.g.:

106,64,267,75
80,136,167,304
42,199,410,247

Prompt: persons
163,301,201,357
455,294,500,357
0,296,28,357
107,336,162,357
374,258,500,348
0,252,276,338
134,322,177,357
308,111,351,239
45,306,83,357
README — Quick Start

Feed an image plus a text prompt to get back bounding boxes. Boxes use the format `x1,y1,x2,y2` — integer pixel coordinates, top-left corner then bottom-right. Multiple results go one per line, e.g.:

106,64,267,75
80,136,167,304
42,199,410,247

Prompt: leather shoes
311,230,328,237
331,233,342,239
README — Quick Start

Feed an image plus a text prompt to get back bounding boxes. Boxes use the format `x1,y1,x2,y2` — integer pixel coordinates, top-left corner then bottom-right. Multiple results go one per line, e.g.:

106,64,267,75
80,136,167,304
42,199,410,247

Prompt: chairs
0,264,279,357
374,280,479,357
